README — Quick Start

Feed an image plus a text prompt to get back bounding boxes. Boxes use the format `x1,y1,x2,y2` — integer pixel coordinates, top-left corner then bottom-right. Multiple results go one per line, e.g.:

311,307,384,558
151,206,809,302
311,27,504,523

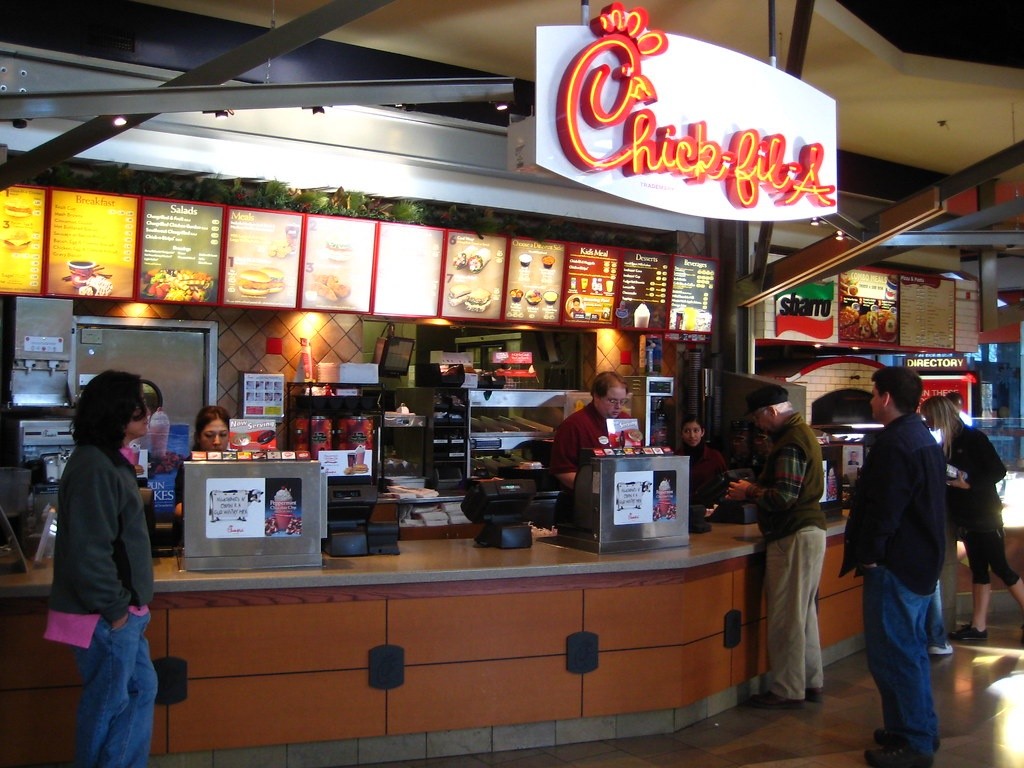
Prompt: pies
453,246,491,273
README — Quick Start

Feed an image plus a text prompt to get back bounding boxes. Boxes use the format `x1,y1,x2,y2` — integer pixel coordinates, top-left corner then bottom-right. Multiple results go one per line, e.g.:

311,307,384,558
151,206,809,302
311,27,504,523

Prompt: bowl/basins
509,288,523,302
525,289,542,305
542,255,555,268
518,253,532,267
543,291,559,304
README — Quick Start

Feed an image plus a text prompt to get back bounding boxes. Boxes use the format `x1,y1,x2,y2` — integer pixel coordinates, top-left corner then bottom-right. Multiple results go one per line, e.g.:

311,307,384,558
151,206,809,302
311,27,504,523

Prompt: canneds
675,312,684,330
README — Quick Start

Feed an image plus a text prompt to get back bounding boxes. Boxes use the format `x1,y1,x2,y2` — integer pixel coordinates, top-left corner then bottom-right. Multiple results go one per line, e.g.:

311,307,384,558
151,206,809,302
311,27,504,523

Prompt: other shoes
928,642,953,655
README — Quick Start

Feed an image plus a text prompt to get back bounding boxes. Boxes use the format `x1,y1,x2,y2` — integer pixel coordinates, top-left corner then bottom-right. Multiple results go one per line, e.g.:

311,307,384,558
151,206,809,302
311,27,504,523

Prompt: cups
356,452,365,465
632,313,650,328
884,278,897,301
348,453,357,467
68,261,95,289
274,500,291,531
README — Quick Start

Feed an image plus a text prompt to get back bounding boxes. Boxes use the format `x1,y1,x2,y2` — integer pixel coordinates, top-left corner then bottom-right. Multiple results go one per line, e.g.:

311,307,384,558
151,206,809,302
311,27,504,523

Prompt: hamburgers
238,268,285,295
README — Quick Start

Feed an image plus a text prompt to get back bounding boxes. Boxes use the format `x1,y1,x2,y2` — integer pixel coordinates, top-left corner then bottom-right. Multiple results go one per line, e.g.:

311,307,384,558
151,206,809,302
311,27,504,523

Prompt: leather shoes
805,687,824,701
751,690,804,709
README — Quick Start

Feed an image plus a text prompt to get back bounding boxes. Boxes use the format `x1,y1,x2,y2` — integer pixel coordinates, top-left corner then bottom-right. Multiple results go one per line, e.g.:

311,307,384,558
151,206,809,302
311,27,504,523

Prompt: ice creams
275,485,293,529
658,480,672,516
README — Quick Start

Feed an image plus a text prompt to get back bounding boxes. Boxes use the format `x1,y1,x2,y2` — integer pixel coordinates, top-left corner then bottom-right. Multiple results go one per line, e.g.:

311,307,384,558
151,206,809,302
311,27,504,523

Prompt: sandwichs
448,284,492,314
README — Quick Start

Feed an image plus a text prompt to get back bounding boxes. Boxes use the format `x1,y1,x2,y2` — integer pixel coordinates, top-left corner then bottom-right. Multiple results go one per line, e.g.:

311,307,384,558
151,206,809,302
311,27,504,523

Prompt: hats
743,383,788,416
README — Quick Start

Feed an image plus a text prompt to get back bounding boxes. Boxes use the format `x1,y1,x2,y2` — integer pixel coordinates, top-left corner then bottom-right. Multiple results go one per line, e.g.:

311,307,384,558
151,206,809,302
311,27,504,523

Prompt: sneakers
863,746,933,768
1021,624,1024,644
874,726,940,751
947,621,988,640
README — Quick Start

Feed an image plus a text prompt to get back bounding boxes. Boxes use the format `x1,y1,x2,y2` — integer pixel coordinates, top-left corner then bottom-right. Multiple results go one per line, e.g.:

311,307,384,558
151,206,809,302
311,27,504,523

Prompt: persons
545,368,632,491
673,413,728,498
170,404,231,546
927,579,953,654
724,387,830,710
838,362,949,768
919,395,1024,644
945,392,964,413
44,368,156,768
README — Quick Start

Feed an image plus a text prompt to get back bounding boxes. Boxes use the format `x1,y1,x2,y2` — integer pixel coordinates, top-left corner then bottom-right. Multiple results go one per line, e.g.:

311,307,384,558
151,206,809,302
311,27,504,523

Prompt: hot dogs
3,205,32,217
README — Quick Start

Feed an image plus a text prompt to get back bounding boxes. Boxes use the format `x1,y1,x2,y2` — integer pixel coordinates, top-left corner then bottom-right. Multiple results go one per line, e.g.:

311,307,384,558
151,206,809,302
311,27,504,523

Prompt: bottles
147,406,170,458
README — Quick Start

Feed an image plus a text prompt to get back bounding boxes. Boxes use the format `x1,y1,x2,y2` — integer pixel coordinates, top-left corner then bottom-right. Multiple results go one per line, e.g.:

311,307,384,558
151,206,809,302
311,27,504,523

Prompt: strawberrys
148,282,170,298
153,450,186,474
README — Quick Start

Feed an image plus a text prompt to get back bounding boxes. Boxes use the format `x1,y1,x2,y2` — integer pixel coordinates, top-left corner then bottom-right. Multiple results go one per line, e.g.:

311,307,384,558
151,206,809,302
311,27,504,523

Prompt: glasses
752,407,769,424
598,396,629,407
